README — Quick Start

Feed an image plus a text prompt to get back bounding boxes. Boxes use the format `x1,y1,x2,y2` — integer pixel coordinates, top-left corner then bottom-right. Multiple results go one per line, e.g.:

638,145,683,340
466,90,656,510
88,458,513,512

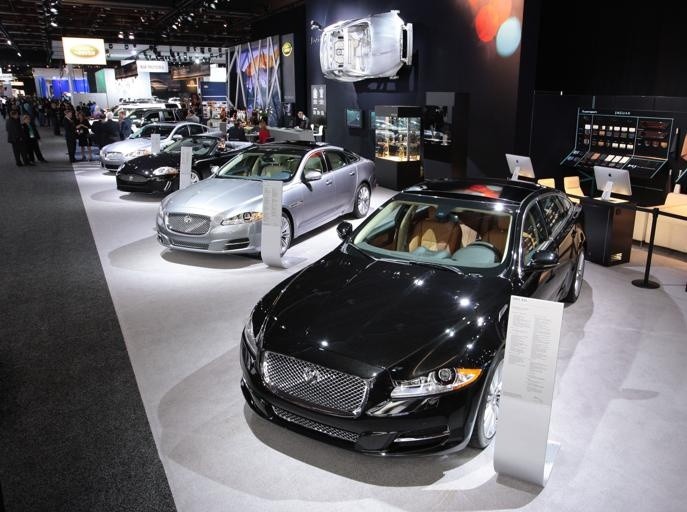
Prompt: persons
179,93,203,124
226,121,249,142
212,109,219,119
219,106,228,122
230,112,237,121
258,121,270,144
249,110,260,126
0,93,133,167
297,111,310,129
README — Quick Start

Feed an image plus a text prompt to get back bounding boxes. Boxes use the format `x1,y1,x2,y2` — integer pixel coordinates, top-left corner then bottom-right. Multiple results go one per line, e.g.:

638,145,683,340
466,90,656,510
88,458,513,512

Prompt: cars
157,137,376,259
115,134,254,195
127,108,185,132
100,120,220,171
240,175,587,458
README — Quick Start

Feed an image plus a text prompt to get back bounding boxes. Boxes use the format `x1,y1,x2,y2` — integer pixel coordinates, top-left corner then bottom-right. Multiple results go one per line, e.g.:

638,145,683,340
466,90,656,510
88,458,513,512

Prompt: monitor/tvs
344,108,364,129
593,166,633,204
505,154,537,184
368,110,375,131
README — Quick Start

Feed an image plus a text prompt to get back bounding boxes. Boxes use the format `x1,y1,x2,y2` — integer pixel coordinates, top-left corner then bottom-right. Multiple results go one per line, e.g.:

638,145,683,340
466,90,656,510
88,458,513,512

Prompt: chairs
536,174,586,204
261,156,289,179
406,205,510,259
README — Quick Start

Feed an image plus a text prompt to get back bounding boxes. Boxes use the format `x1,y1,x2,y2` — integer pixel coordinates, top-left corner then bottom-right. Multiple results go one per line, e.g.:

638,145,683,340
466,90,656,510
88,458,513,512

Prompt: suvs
76,99,181,135
